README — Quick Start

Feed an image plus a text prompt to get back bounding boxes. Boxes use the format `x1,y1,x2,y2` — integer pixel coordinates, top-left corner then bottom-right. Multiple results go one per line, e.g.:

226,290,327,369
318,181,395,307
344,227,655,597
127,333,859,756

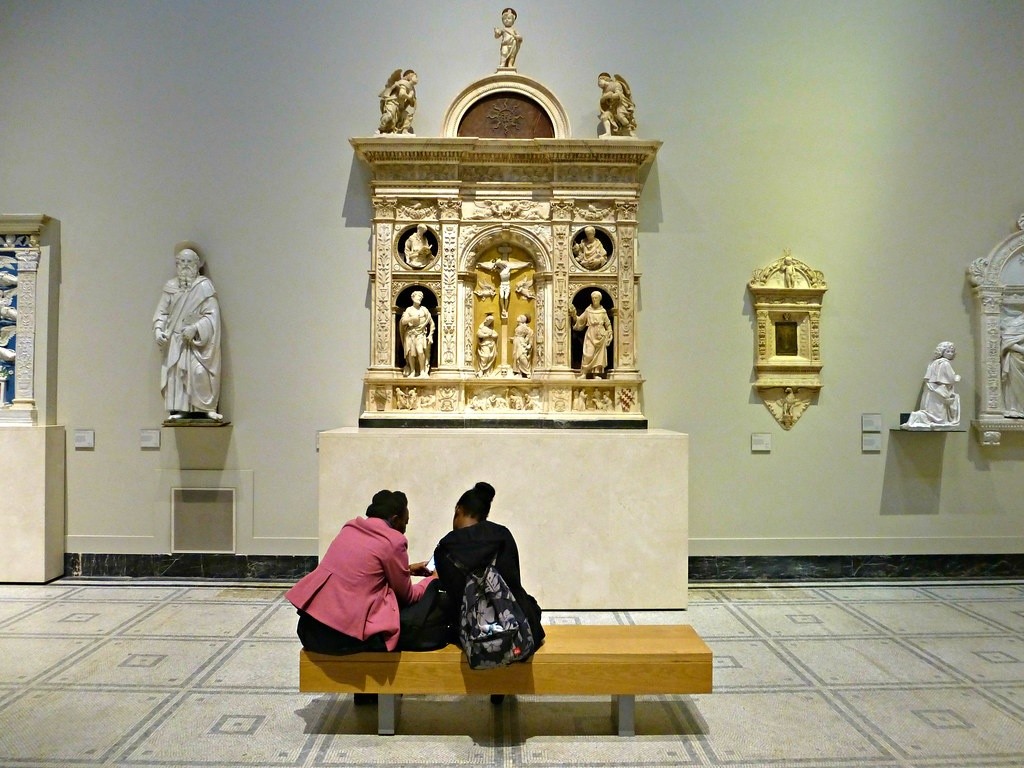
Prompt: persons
494,8,523,68
434,481,546,705
574,226,607,270
900,340,961,429
477,258,534,378
780,256,796,288
152,240,224,421
1002,309,1024,418
569,290,614,379
399,291,435,378
405,223,434,268
284,490,438,708
373,69,418,134
395,386,613,411
597,72,637,138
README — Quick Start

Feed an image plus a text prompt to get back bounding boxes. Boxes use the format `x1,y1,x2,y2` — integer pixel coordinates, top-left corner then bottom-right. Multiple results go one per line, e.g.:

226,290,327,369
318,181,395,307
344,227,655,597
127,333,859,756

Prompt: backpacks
437,545,535,670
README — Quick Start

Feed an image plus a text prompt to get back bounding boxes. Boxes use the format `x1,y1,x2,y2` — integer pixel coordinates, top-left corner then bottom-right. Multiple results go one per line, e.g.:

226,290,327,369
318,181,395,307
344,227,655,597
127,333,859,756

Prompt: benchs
293,623,715,734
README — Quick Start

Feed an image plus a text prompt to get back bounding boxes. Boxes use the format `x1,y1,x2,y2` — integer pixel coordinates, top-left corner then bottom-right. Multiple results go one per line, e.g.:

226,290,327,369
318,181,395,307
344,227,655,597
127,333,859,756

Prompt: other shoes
354,693,378,706
490,694,504,705
